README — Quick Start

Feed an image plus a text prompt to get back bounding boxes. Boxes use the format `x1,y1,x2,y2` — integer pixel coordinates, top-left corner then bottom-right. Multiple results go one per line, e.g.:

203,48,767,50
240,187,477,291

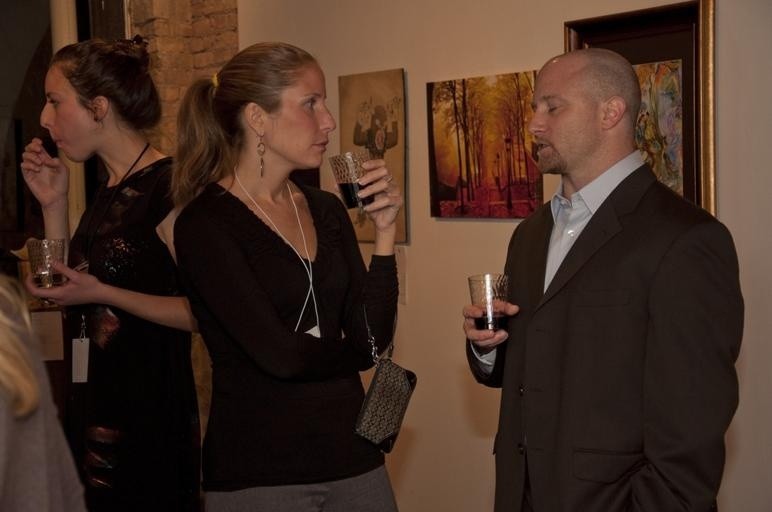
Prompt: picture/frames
563,0,717,227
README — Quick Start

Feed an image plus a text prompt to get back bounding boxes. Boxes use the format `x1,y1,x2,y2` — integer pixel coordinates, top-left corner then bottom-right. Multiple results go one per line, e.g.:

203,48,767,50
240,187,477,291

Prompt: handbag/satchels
353,359,417,453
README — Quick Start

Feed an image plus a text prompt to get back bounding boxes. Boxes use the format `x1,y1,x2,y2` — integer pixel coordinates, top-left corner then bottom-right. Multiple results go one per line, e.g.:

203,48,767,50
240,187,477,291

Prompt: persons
462,48,745,510
1,275,88,511
19,32,201,511
171,43,405,509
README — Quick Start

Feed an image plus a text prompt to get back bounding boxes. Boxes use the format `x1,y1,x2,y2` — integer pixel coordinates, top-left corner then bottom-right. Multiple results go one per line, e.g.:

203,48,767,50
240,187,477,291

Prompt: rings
386,175,393,182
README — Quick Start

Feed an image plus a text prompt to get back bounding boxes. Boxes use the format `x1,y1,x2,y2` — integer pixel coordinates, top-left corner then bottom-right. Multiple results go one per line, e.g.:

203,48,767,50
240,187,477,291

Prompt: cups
467,271,509,337
28,235,66,287
329,147,374,209
